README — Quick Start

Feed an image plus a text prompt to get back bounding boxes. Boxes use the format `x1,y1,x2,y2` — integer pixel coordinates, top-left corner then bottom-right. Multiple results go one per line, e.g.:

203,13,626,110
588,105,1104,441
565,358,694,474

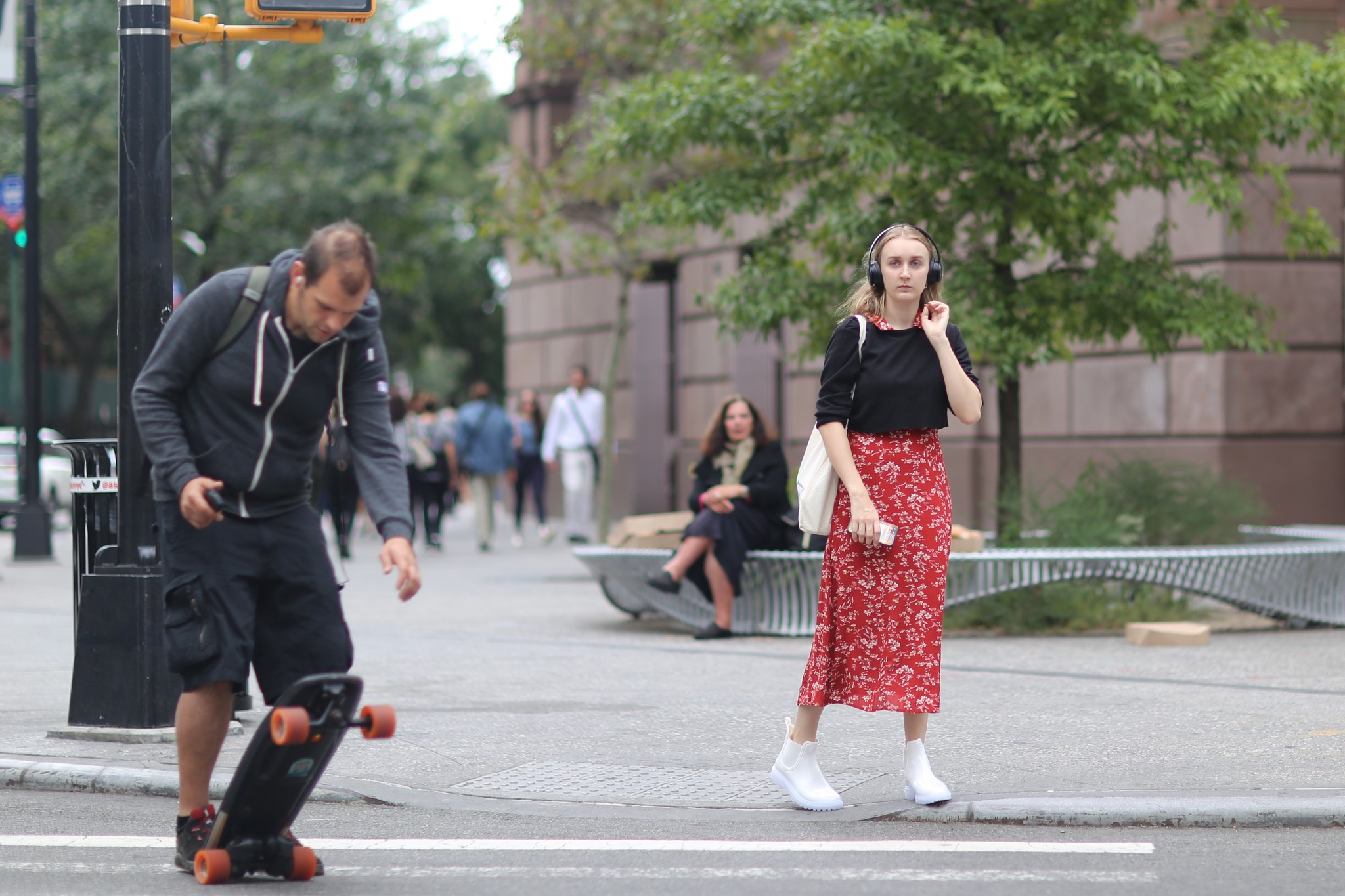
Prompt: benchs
574,518,1345,632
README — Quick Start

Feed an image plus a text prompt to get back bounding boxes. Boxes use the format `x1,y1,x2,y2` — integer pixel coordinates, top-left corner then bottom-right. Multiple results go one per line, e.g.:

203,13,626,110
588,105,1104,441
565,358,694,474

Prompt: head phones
866,224,943,288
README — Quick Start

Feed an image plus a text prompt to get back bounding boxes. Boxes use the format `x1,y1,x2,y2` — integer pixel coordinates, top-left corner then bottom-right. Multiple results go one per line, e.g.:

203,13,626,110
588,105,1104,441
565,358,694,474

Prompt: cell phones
846,516,899,546
205,488,225,512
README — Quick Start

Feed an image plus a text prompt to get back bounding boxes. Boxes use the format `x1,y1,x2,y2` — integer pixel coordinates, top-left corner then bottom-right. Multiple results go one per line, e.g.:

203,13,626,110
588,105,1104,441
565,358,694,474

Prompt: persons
131,220,422,878
539,363,606,546
642,393,790,640
450,381,513,551
508,384,552,546
317,397,359,558
769,222,984,811
389,387,461,547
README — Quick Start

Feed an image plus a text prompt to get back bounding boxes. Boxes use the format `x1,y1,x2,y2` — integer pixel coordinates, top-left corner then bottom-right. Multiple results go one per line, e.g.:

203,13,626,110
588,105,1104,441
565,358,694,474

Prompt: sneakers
284,828,324,875
176,805,218,873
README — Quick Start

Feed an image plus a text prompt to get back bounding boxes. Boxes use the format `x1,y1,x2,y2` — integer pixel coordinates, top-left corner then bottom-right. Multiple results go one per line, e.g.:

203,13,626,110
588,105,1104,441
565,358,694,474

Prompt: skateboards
194,673,395,883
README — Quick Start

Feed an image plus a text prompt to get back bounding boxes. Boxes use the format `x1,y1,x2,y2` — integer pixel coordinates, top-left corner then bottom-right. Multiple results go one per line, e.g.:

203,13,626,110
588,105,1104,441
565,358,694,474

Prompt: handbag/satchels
796,417,848,535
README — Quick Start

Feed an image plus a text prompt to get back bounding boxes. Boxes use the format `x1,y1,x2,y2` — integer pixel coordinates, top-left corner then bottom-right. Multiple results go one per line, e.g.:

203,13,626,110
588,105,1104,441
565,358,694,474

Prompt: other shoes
695,621,731,640
645,571,680,595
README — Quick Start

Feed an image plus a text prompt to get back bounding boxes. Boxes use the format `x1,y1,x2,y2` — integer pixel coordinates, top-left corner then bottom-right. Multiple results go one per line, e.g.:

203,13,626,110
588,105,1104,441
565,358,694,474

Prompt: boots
903,739,951,805
771,716,843,811
